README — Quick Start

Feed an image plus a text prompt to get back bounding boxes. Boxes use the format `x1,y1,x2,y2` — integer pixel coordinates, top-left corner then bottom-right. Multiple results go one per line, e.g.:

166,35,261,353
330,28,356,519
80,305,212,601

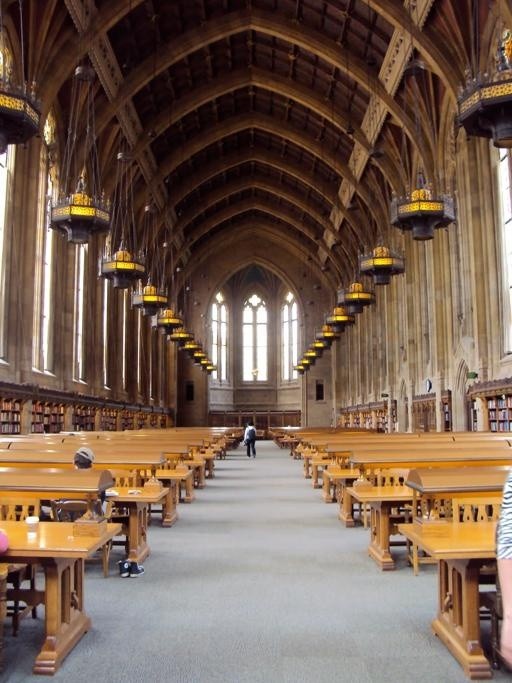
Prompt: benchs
267,426,511,679
1,427,244,675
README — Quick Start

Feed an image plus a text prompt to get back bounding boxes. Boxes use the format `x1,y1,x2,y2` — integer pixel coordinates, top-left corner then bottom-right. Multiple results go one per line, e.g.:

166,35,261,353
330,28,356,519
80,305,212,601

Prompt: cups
25,515,39,543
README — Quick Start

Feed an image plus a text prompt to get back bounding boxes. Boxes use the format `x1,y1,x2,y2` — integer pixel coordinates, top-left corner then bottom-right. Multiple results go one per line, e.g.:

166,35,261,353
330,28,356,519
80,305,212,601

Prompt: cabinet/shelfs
0,382,97,438
464,378,512,434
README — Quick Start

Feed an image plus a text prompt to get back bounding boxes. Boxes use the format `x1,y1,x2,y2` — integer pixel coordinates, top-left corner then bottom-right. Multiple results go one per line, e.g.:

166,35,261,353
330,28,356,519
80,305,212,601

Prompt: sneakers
130,562,144,577
118,561,130,577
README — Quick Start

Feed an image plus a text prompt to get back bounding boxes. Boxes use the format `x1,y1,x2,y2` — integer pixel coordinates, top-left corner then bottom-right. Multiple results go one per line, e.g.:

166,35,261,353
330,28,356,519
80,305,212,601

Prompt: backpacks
249,430,255,440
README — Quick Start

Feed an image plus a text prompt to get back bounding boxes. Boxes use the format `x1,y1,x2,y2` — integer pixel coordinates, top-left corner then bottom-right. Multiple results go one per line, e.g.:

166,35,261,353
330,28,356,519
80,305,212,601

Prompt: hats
75,448,94,469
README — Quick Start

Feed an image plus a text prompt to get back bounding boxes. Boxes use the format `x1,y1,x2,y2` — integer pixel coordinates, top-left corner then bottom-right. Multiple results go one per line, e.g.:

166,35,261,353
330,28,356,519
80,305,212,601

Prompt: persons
244,420,257,458
494,471,512,665
0,528,9,553
49,447,115,513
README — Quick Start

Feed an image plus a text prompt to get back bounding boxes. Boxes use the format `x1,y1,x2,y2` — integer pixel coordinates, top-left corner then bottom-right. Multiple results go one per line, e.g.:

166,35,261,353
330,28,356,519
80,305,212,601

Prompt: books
0,397,167,434
486,396,512,432
343,410,395,433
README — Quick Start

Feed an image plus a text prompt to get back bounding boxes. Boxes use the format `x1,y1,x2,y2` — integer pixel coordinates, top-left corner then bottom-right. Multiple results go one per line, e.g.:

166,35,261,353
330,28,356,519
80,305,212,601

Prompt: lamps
448,3,511,156
94,151,140,293
356,243,407,287
47,63,114,245
384,2,461,242
292,313,340,375
128,277,216,374
1,0,46,164
334,282,380,315
323,306,356,329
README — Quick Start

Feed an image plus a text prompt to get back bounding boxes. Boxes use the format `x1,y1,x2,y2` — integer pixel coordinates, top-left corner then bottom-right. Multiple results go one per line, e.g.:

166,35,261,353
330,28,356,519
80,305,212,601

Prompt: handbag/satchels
244,441,247,446
